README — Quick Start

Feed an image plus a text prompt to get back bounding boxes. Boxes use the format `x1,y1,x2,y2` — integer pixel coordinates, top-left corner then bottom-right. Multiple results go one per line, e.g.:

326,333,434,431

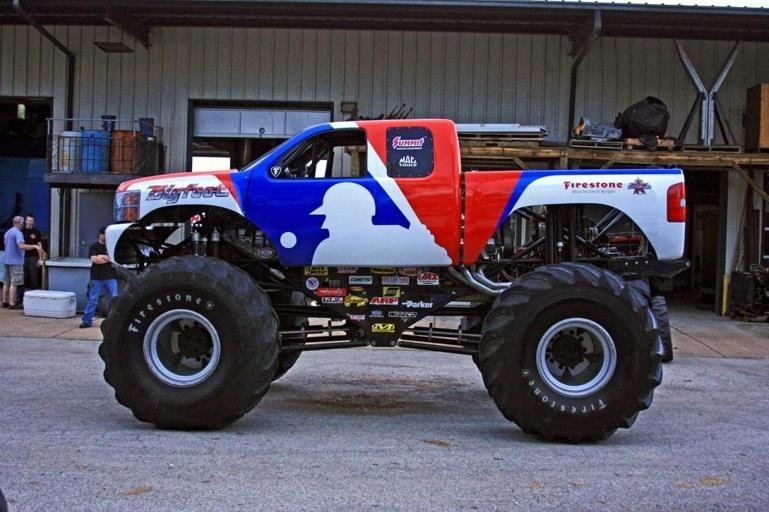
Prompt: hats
98,226,106,236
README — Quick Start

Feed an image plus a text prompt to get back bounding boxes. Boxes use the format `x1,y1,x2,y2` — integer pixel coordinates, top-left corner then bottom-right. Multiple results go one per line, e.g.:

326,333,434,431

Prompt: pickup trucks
98,119,690,444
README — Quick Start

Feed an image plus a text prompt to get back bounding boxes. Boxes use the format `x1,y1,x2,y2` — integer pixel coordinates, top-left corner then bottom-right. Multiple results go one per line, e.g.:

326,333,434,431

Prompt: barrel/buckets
47,130,141,174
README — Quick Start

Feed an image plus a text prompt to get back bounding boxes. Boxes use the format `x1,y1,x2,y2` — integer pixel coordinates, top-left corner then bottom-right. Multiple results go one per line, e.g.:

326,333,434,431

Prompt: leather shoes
8,304,23,309
3,303,8,308
79,321,88,328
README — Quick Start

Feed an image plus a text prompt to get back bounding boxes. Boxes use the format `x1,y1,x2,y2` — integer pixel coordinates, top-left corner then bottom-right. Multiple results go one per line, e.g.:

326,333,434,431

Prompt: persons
16,213,43,305
2,216,41,310
78,225,118,328
